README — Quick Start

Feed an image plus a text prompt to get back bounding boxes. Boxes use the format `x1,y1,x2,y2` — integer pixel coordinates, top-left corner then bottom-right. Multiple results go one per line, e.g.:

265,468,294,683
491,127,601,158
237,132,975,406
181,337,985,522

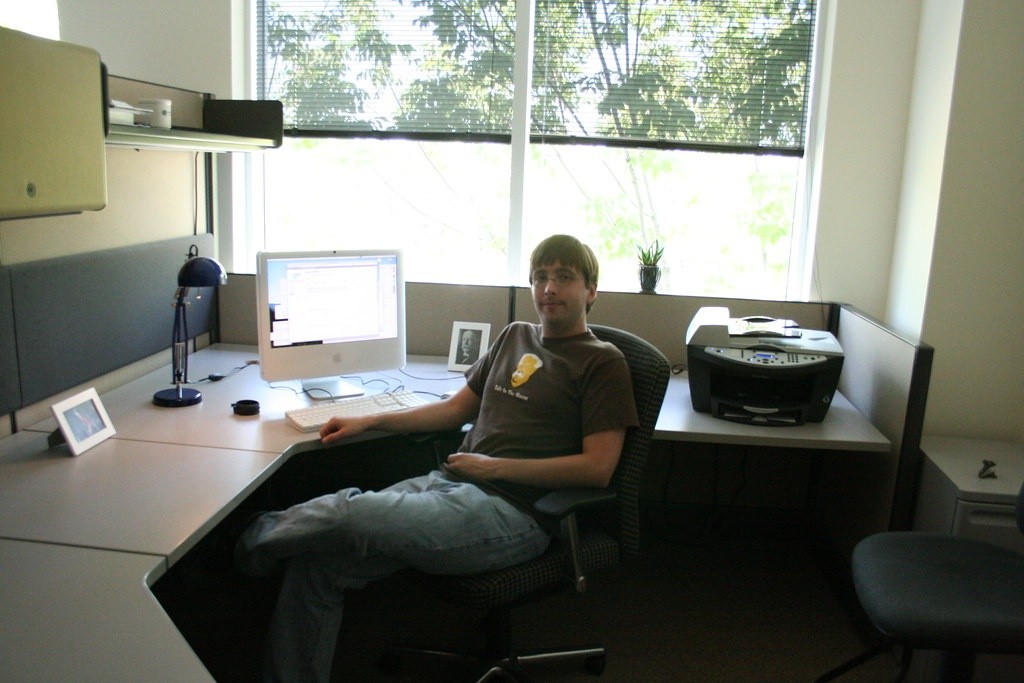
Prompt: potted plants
634,241,664,295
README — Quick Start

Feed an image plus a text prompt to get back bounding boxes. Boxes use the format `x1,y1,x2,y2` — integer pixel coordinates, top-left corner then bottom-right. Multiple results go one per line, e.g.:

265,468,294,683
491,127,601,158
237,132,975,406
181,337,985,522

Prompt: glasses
531,272,583,288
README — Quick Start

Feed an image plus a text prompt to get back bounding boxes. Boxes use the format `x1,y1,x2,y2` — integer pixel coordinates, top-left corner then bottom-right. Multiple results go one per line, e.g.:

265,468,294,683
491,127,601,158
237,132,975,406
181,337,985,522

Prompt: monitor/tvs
255,249,407,399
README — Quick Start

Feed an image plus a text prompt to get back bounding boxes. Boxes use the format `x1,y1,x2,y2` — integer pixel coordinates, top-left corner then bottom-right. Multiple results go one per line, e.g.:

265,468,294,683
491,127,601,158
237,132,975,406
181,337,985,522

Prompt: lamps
152,245,228,408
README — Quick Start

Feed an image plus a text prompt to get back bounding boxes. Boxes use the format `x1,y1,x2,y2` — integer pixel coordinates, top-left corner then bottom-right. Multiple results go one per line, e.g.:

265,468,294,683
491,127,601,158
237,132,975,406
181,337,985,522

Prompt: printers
684,305,845,428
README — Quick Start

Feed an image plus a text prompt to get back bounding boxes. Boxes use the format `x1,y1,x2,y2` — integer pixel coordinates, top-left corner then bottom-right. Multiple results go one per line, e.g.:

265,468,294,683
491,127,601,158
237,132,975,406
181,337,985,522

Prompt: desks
0,336,893,683
894,437,1024,683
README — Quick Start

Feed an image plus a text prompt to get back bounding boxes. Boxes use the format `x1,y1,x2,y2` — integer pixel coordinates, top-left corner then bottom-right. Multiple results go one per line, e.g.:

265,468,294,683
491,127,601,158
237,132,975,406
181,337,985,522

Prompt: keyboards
285,391,429,432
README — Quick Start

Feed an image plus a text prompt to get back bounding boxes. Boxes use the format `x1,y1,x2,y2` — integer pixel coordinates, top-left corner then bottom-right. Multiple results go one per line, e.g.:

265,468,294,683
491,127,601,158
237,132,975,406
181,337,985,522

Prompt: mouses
440,390,457,401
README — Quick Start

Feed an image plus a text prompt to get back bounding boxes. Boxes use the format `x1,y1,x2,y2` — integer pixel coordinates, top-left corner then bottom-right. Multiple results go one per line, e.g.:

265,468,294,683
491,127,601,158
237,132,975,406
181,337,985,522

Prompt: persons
227,234,640,683
457,330,480,364
74,409,99,436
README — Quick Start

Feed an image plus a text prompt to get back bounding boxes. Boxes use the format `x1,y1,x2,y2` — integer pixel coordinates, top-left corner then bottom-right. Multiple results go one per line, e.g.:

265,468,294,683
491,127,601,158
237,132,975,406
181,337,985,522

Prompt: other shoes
180,509,264,563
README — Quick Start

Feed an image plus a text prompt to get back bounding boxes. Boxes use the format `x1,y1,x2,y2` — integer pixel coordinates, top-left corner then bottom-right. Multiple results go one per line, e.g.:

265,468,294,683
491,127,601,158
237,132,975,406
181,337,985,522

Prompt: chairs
366,319,669,683
849,482,1024,683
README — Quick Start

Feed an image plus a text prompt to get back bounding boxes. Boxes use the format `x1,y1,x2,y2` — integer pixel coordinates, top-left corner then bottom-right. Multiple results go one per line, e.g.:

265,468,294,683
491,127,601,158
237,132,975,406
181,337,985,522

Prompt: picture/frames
447,320,492,372
46,387,117,457
